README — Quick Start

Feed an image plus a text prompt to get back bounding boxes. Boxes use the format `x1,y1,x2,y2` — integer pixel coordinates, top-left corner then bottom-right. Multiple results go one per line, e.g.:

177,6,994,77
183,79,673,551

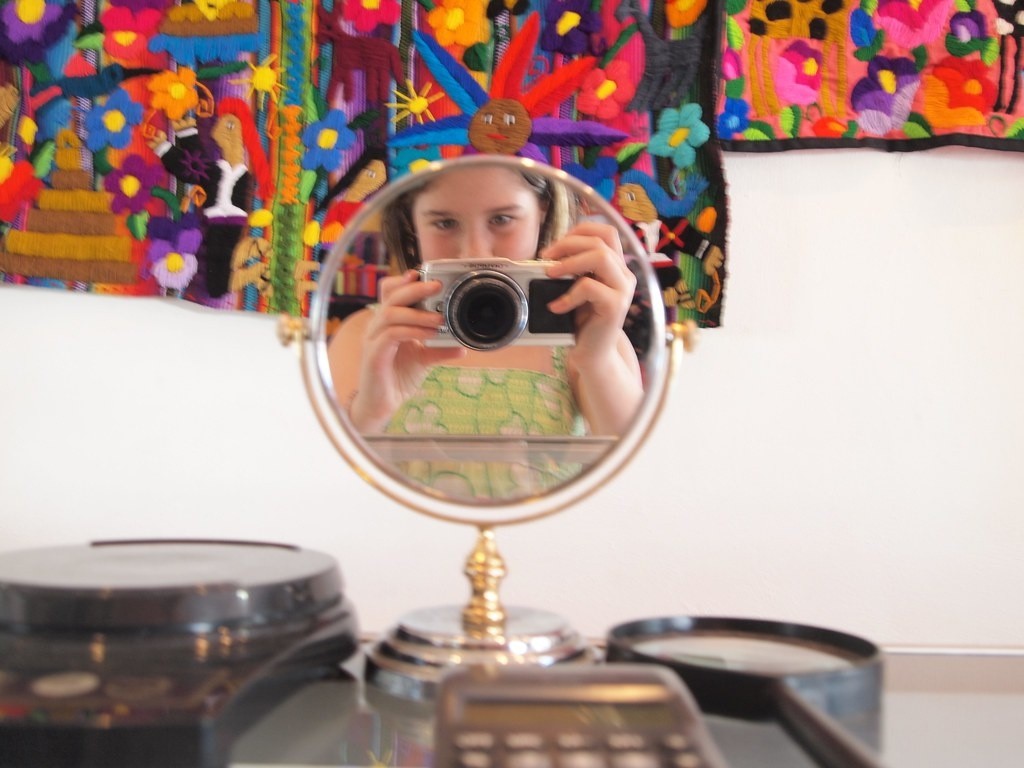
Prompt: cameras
413,257,574,353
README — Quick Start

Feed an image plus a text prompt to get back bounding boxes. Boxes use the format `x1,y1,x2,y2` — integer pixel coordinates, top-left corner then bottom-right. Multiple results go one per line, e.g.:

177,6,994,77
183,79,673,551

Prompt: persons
326,166,646,505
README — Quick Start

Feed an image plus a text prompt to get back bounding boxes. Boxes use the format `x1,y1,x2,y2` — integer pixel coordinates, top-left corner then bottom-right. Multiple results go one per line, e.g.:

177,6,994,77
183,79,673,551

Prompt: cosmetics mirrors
311,153,667,508
